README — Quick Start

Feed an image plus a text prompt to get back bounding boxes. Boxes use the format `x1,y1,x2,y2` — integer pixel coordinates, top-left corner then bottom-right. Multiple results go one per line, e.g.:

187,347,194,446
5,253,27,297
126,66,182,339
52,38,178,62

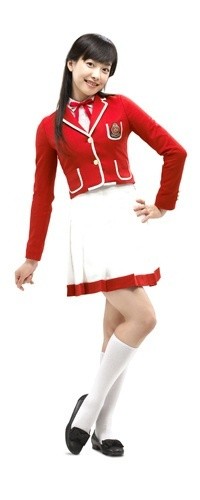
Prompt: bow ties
70,99,93,122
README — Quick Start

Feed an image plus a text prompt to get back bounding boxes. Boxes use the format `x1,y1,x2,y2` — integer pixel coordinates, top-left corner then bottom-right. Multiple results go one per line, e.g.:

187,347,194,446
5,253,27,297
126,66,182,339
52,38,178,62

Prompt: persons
14,34,188,456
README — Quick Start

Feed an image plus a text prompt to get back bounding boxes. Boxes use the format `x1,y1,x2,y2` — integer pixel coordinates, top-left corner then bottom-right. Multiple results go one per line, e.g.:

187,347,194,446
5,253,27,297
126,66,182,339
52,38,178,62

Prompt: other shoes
91,429,124,456
65,394,91,455
